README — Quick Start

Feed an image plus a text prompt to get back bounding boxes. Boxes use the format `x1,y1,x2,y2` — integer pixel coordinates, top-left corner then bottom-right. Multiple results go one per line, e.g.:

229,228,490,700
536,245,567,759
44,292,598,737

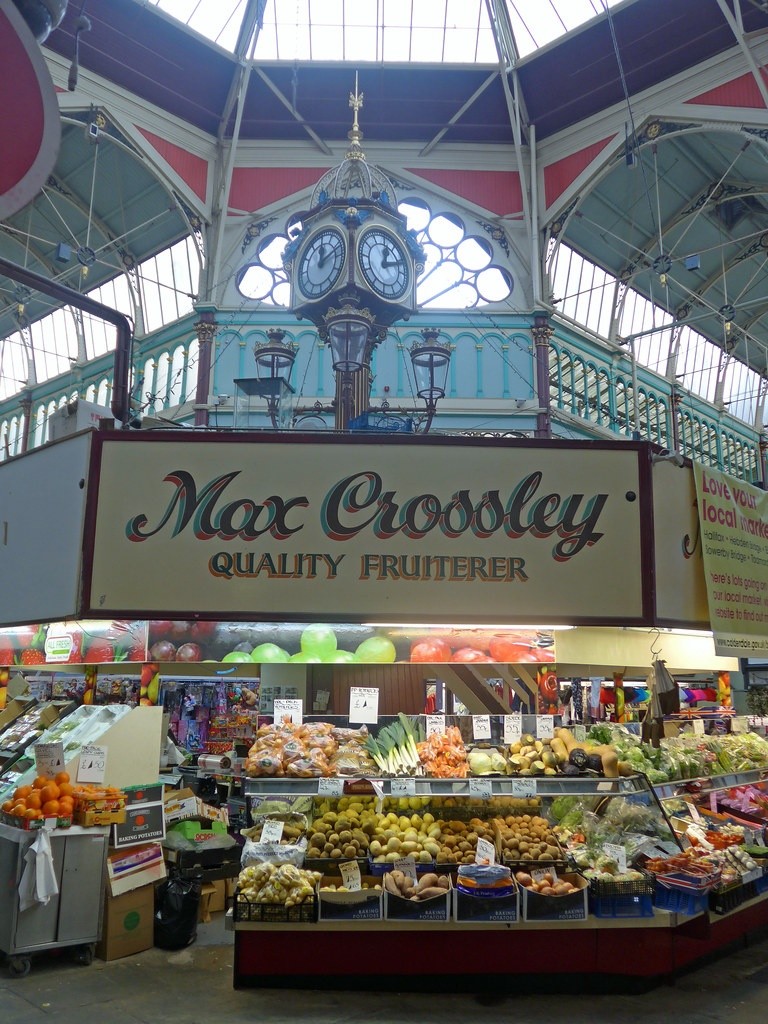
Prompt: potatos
247,810,578,901
312,795,541,816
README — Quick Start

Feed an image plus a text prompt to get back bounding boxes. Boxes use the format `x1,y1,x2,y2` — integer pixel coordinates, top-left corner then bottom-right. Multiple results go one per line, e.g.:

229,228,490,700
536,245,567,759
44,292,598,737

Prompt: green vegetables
549,795,671,865
587,722,768,782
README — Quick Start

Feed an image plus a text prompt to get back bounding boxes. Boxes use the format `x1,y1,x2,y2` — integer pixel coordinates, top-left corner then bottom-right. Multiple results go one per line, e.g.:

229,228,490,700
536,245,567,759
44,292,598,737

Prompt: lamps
250,327,300,429
406,327,456,435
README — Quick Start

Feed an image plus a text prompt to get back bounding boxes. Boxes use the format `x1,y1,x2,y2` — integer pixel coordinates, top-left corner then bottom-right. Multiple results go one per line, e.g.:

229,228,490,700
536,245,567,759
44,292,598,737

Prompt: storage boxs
383,872,451,923
240,797,568,876
48,399,122,441
63,704,132,763
164,796,230,828
25,705,103,752
0,701,78,753
94,884,155,961
578,868,655,919
197,885,217,924
653,872,708,915
515,872,588,923
162,843,243,870
318,876,384,922
0,672,39,733
669,800,768,916
0,750,20,774
232,886,318,925
449,873,520,924
208,879,225,914
0,754,167,897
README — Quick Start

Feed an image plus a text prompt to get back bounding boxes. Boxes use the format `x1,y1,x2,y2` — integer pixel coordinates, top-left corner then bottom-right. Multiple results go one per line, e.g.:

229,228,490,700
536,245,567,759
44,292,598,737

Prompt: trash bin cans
180,766,207,795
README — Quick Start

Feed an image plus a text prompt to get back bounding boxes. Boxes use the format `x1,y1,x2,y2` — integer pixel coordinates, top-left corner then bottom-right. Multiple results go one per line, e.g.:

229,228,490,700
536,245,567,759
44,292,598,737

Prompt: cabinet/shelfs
223,770,710,995
652,767,768,965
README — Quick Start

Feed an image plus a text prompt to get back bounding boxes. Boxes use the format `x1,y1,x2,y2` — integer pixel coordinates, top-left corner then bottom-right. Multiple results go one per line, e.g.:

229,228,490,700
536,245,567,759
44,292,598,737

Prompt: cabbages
575,854,646,894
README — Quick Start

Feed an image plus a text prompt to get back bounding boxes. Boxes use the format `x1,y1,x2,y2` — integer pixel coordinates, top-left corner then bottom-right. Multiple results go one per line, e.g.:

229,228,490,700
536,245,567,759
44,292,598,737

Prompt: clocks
297,228,345,299
358,229,410,300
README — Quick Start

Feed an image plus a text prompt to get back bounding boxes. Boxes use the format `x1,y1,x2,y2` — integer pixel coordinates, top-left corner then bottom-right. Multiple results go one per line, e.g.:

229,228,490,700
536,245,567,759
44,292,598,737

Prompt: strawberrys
0,622,146,666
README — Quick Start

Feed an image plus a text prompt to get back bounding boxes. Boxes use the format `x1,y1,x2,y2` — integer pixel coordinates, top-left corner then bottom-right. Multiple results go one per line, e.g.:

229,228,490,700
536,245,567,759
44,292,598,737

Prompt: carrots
416,725,468,778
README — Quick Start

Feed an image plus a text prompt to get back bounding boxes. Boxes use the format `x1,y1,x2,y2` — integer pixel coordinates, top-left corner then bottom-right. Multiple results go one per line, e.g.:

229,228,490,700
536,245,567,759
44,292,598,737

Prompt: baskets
232,884,318,923
753,868,767,895
697,830,744,850
709,875,756,914
655,872,721,914
589,877,654,917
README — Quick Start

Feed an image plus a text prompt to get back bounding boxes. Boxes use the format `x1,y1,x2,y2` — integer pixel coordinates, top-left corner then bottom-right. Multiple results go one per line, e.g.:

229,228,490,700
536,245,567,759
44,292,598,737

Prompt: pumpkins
510,728,633,779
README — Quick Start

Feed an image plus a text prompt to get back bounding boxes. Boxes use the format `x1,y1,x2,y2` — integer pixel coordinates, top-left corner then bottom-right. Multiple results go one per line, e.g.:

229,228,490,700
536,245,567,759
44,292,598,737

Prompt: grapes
147,618,557,666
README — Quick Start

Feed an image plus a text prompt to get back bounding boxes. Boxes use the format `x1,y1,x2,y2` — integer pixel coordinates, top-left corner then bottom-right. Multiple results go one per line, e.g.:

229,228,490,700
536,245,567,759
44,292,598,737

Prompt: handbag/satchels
18,826,59,912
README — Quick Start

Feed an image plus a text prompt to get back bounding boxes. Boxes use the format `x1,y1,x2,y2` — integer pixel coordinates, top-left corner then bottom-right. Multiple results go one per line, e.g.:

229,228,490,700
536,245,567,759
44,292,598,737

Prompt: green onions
357,712,426,778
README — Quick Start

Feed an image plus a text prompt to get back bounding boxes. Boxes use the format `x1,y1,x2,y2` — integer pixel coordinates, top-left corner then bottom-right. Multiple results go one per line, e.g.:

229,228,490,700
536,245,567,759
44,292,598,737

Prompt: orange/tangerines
2,771,75,818
